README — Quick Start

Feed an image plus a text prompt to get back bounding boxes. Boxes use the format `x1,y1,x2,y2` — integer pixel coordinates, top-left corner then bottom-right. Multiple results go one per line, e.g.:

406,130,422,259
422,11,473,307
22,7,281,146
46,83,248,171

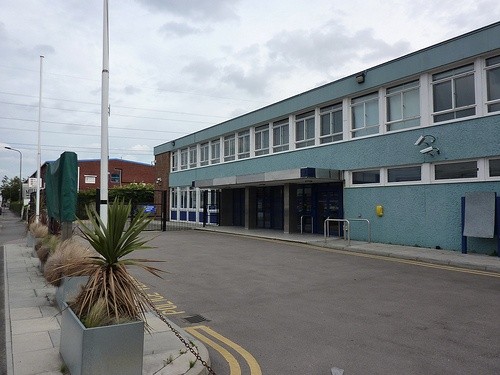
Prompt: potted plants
59,197,171,375
28,224,100,314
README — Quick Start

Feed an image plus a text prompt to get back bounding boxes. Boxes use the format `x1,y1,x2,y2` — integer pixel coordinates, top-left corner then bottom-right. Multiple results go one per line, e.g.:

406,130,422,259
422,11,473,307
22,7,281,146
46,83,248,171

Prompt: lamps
356,73,365,84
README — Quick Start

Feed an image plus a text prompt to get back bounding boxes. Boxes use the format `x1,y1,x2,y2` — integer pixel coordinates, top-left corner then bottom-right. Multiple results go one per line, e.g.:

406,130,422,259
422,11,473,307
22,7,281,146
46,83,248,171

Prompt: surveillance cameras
156,178,161,184
415,134,440,156
